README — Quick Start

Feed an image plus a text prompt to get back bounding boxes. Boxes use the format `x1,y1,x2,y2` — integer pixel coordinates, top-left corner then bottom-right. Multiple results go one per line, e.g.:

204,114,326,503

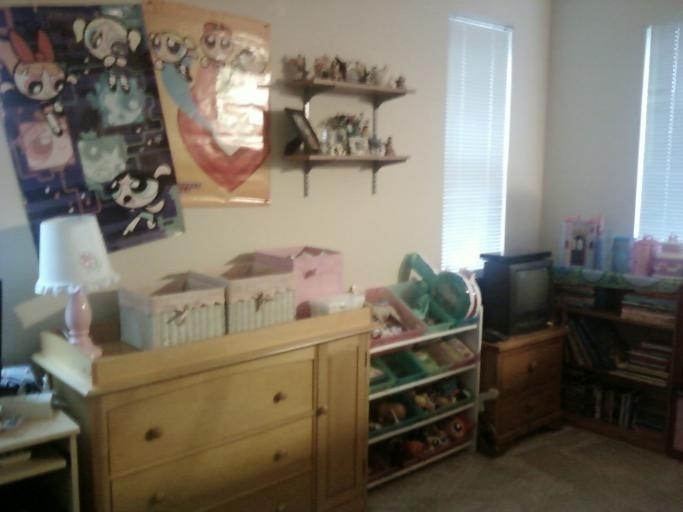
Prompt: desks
0,410,80,512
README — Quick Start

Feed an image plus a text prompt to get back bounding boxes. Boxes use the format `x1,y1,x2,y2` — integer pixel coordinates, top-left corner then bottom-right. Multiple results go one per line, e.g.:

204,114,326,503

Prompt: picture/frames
286,109,323,153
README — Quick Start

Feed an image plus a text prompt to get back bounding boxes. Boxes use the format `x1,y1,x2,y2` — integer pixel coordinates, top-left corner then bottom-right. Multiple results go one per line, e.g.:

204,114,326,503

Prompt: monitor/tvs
484,259,556,336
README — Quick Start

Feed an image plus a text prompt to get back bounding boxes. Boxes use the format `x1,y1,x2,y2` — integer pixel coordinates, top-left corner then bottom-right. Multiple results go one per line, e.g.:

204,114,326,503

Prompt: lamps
34,214,122,359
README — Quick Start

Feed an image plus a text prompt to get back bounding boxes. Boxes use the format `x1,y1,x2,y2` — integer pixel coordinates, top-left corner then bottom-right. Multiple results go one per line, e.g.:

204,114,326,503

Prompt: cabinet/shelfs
480,327,570,445
284,78,417,197
557,267,683,455
32,308,317,512
367,306,483,490
315,333,367,512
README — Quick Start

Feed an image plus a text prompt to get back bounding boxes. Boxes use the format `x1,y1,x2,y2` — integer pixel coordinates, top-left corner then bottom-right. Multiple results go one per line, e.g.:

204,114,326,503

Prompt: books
559,286,676,444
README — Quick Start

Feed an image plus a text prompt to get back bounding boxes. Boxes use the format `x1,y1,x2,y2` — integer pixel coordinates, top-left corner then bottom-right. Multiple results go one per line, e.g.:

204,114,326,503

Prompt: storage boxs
254,246,344,316
